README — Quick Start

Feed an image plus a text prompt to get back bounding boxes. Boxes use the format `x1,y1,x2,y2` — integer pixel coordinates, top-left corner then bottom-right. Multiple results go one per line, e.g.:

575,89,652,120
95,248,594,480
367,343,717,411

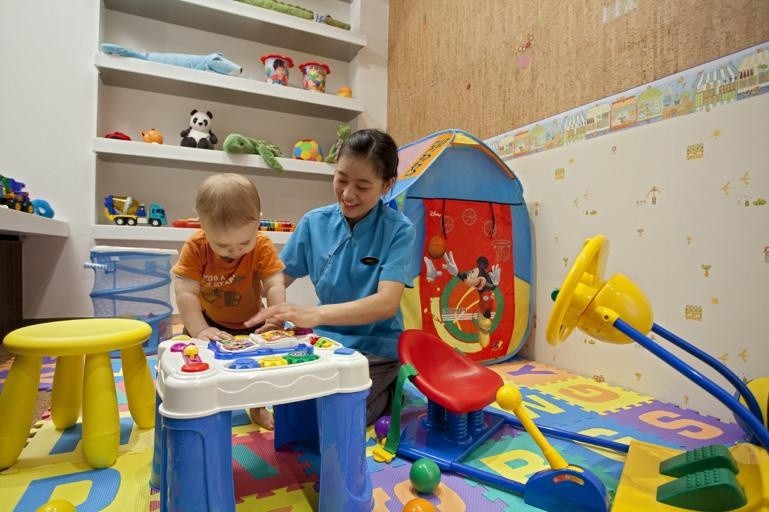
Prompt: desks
142,319,375,512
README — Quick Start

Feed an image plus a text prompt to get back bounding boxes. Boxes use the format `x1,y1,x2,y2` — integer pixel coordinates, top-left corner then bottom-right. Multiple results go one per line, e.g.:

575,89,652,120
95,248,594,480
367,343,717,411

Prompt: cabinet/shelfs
94,3,362,244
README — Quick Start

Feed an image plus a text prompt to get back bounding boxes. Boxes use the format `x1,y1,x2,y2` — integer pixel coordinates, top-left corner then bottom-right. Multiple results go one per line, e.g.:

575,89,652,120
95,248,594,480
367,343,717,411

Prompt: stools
1,316,158,473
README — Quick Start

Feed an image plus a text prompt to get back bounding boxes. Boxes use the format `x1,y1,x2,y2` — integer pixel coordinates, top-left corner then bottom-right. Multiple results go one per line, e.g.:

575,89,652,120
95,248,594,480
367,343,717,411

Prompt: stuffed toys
227,134,290,172
142,128,166,145
105,130,133,141
180,108,218,149
97,42,244,81
1,176,57,218
294,138,323,162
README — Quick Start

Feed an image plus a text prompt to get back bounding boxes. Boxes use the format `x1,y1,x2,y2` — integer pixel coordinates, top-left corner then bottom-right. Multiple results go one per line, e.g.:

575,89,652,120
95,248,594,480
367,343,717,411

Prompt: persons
242,128,418,437
171,167,287,432
